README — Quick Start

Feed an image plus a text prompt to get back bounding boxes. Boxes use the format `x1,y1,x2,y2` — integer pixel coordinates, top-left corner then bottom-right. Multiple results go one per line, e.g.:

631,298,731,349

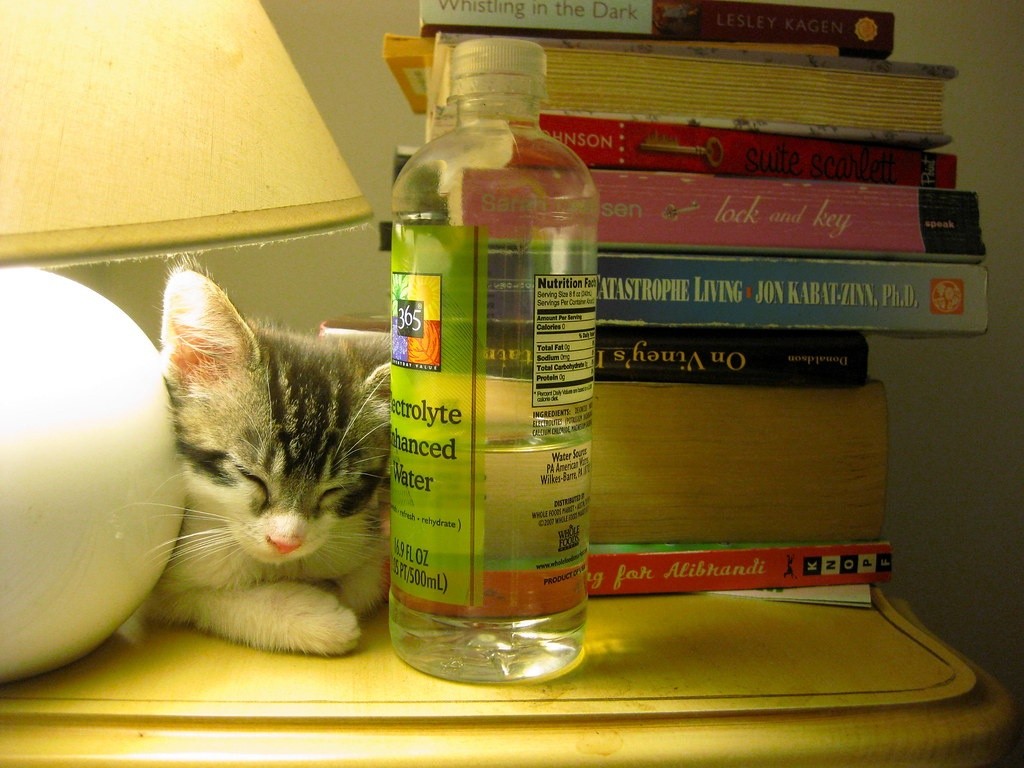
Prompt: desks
0,588,1018,767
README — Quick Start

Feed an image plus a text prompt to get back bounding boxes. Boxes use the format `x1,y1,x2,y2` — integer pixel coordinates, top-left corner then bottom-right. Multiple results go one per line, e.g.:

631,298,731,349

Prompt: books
320,0,990,602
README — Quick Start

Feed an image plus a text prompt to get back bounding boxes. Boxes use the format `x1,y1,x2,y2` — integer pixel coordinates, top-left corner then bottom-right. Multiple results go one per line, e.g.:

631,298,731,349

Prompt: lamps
0,1,377,688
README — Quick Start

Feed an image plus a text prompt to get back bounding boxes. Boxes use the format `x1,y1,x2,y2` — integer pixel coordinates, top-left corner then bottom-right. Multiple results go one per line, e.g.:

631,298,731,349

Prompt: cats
152,259,397,657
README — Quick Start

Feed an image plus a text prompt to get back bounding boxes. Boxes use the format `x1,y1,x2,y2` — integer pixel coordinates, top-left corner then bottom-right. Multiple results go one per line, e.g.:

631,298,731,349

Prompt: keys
639,135,724,168
665,200,701,222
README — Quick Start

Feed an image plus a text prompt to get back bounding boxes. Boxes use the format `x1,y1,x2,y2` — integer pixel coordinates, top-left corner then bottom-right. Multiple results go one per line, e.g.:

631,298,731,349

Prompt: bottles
387,37,601,685
